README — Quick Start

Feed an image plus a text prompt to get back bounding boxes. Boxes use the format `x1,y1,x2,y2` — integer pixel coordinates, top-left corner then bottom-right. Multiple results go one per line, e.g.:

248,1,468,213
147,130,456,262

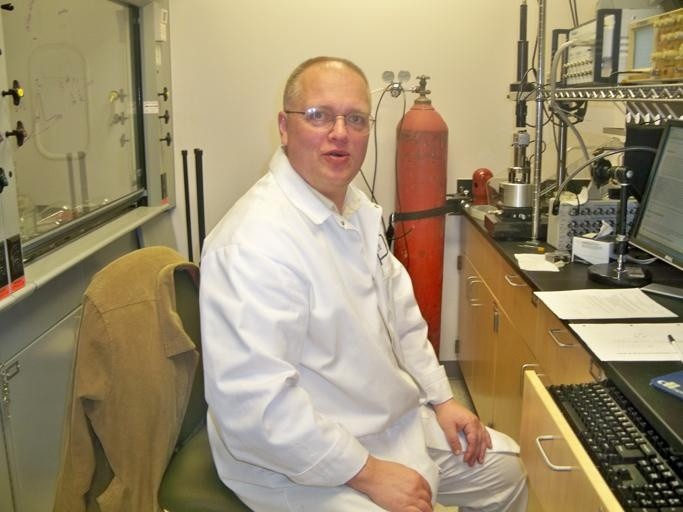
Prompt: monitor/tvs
628,119,683,301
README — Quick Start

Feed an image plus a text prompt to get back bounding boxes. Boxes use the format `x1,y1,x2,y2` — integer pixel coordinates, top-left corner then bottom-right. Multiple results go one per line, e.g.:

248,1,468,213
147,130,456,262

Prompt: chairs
81,243,250,511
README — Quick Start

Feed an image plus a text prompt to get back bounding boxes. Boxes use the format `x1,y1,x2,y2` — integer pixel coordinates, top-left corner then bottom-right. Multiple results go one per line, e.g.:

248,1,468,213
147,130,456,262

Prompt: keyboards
546,377,683,512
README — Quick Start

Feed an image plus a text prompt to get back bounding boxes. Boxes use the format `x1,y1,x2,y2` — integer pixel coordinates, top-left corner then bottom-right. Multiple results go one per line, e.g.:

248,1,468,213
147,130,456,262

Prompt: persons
199,56,529,512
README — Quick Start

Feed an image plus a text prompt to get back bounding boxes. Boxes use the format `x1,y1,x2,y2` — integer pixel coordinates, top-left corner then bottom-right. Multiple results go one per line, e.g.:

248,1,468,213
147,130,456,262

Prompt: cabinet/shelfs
457,218,682,511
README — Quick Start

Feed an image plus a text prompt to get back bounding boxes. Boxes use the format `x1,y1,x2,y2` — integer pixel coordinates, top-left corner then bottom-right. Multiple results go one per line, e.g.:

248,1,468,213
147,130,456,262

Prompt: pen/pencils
517,245,545,252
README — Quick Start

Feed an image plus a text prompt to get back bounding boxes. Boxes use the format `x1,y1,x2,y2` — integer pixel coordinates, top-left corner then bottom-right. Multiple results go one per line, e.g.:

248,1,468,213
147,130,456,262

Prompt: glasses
284,108,377,134
